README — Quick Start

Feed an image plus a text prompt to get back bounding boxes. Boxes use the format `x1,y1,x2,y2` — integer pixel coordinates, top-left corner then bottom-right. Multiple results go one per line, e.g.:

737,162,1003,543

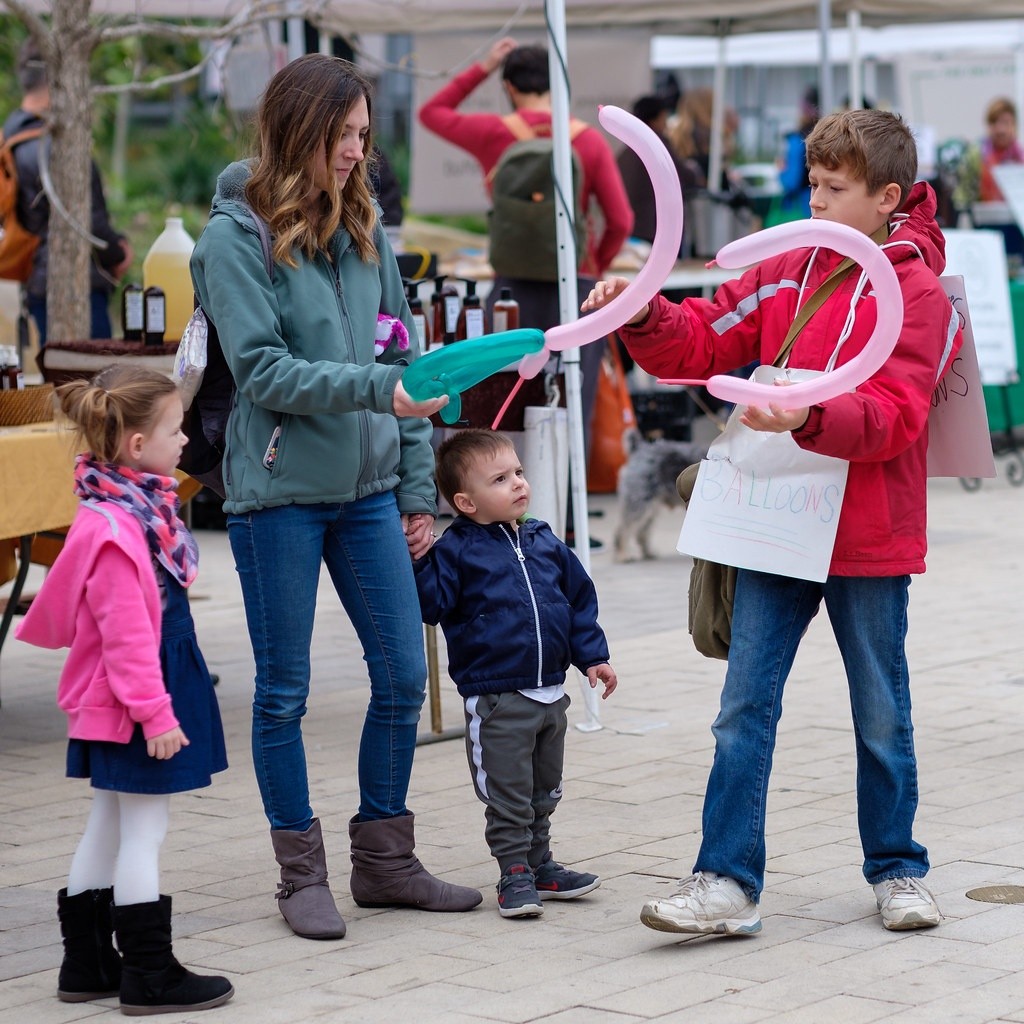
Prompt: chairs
0,276,26,388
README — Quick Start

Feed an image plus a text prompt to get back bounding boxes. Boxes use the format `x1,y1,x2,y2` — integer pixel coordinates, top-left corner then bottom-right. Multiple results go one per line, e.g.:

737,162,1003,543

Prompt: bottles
409,297,430,353
492,287,520,333
441,285,460,346
455,294,489,341
121,282,145,343
0,354,24,391
141,216,196,342
143,285,167,346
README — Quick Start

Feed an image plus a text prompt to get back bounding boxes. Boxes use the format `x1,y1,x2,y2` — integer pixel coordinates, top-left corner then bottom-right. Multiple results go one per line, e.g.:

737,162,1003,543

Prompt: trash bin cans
959,277,1024,493
692,195,735,260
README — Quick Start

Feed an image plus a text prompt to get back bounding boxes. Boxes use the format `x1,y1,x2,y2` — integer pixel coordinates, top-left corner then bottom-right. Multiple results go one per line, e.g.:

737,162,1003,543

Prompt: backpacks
0,123,49,281
488,114,586,279
161,199,275,499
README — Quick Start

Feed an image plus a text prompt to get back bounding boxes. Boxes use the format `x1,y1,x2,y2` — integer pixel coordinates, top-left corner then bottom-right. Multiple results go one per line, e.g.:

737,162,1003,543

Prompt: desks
0,416,195,655
35,341,586,752
597,259,758,303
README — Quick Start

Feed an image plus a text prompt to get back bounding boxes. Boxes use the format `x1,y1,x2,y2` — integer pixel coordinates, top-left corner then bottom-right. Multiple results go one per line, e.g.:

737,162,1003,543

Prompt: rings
431,532,437,537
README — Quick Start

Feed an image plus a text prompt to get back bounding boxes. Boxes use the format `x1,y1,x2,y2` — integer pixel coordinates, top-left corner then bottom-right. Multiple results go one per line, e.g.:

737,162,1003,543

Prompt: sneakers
639,869,764,936
530,852,600,900
873,873,943,932
495,861,542,917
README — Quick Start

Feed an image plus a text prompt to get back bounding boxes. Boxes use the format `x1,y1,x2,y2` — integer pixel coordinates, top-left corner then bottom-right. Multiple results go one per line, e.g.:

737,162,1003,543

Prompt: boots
54,886,127,1002
268,817,348,940
348,810,483,912
111,894,233,1013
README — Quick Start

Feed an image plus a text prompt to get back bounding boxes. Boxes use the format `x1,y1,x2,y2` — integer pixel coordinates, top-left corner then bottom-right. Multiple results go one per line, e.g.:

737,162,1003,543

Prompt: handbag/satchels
679,461,736,659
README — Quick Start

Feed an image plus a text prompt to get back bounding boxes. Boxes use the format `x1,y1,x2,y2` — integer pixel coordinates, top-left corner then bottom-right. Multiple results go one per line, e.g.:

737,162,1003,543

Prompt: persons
578,106,965,937
974,100,1024,201
364,142,403,230
408,429,618,916
416,36,636,472
0,32,132,361
13,363,234,1016
619,94,772,258
179,53,490,941
776,88,817,217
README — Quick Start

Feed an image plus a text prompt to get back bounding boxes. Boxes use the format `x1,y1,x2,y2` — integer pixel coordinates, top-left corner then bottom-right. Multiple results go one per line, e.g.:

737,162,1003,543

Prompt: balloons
400,102,685,425
656,218,906,413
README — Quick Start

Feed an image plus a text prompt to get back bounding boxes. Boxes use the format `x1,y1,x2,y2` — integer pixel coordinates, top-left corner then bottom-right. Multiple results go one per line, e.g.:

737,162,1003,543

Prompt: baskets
0,382,55,428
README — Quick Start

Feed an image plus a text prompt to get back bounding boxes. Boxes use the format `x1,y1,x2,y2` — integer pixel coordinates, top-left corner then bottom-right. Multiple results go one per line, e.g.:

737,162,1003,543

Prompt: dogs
614,427,711,564
